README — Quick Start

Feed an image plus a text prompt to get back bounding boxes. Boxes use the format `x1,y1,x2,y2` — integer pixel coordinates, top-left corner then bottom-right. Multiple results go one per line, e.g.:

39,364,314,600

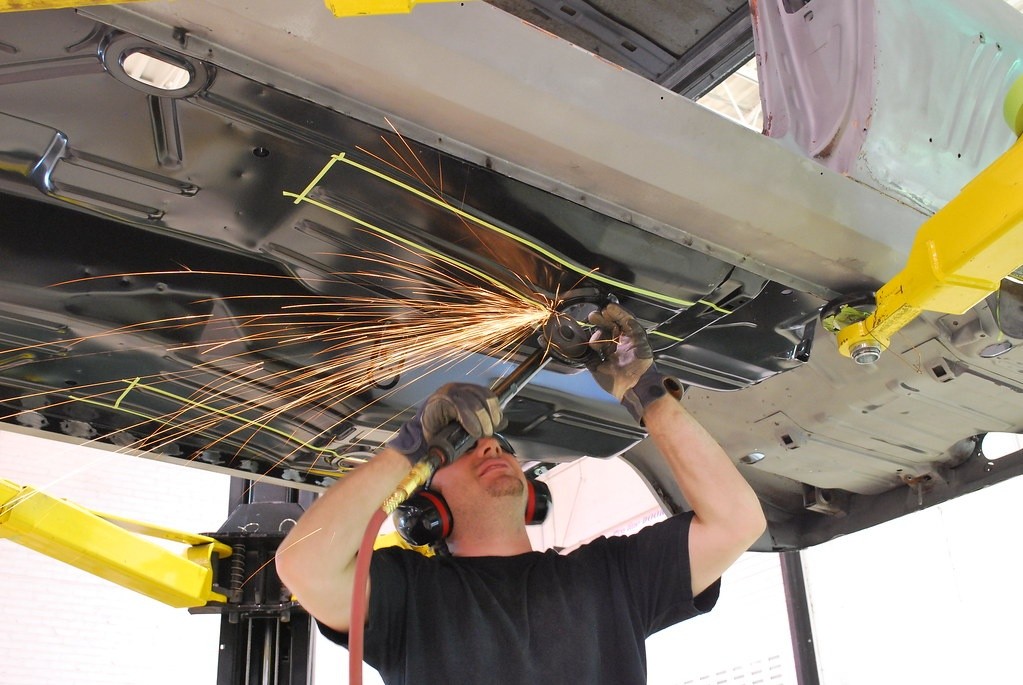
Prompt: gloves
584,302,684,427
388,382,508,467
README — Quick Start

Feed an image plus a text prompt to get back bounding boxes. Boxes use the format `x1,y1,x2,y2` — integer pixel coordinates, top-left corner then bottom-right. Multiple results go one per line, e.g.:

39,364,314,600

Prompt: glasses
465,432,516,456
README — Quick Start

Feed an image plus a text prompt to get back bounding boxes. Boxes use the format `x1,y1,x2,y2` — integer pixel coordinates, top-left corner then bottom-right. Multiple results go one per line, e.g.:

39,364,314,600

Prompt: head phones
392,475,552,549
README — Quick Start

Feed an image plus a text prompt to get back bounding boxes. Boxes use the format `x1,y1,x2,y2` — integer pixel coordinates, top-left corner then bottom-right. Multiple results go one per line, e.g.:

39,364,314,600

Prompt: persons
275,304,767,685
